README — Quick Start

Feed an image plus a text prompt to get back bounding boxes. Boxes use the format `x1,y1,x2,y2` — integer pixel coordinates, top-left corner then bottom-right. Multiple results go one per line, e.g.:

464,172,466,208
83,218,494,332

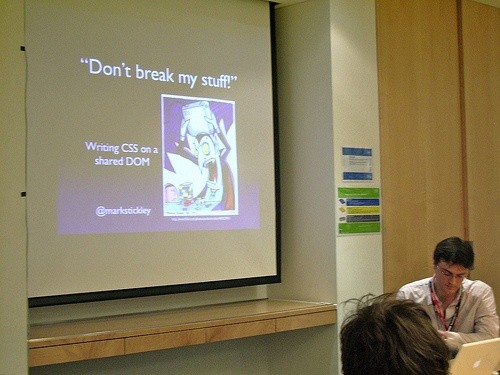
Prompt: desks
28,300,337,368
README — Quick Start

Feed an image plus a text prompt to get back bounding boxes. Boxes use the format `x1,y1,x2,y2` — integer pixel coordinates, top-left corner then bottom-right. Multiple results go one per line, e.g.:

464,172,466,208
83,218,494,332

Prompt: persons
396,236,499,356
338,289,451,375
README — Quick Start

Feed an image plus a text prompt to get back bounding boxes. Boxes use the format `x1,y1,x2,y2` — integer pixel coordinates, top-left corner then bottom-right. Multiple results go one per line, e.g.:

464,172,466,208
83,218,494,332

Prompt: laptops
447,338,500,375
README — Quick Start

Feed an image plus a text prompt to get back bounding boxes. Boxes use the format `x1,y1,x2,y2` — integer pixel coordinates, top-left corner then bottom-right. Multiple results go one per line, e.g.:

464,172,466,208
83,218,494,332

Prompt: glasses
436,266,468,281
